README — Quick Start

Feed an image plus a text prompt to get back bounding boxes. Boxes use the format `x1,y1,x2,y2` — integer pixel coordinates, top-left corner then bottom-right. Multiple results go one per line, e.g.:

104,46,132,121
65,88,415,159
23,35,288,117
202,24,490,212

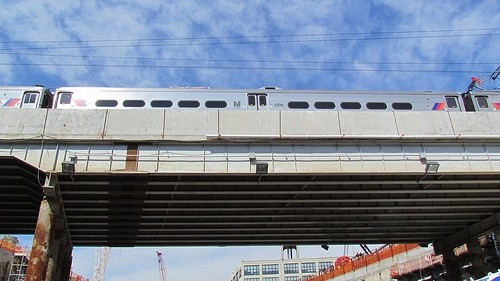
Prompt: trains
0,75,500,112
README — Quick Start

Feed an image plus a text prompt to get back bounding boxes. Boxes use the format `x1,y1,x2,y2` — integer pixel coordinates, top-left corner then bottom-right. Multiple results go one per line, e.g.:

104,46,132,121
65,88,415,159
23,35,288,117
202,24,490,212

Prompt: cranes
155,251,168,281
